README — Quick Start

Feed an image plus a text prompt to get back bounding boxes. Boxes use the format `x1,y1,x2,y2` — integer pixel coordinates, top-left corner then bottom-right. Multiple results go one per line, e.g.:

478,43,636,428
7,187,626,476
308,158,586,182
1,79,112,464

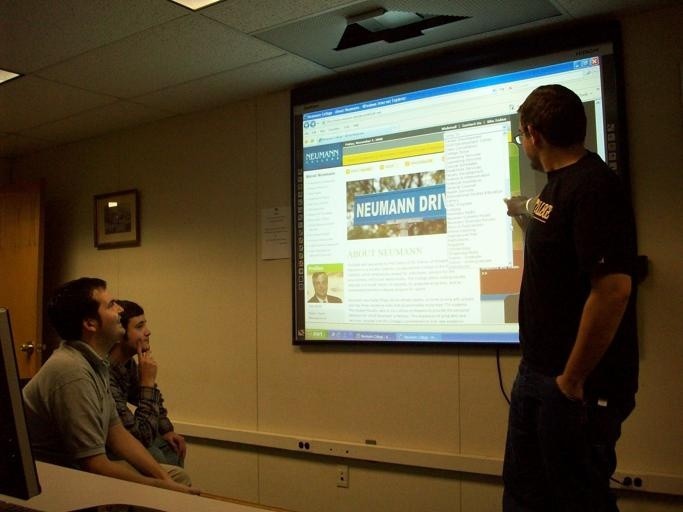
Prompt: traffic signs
335,462,349,487
296,437,311,452
621,471,648,492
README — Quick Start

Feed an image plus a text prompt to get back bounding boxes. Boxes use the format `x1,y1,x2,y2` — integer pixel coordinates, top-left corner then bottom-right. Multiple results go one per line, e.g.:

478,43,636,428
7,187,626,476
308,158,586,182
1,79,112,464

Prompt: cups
514,130,528,145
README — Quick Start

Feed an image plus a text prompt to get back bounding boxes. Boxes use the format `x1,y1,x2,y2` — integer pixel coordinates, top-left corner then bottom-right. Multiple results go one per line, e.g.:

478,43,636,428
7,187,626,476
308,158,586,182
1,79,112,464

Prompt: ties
0,308,42,501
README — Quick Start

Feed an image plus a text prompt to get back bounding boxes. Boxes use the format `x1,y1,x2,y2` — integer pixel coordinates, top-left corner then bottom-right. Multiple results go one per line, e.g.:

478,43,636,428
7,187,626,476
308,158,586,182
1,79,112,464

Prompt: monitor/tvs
0,306,41,502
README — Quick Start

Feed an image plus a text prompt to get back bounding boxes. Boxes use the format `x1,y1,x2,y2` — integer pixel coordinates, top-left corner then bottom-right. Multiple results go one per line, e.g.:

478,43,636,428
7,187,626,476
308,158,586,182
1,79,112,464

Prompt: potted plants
92,189,140,248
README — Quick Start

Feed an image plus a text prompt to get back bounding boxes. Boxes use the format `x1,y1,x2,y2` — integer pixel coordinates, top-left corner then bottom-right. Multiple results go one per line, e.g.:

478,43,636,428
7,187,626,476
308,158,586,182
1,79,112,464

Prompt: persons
501,85,639,510
306,270,341,303
104,297,187,467
20,276,205,496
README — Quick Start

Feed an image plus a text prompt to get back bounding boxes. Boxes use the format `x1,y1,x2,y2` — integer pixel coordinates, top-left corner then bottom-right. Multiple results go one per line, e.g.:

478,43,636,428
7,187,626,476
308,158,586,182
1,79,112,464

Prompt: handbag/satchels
524,197,533,211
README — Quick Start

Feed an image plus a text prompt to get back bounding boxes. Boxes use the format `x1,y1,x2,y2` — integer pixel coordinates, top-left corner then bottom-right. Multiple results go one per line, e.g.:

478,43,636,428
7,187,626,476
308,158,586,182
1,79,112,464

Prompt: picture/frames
0,456,293,512
